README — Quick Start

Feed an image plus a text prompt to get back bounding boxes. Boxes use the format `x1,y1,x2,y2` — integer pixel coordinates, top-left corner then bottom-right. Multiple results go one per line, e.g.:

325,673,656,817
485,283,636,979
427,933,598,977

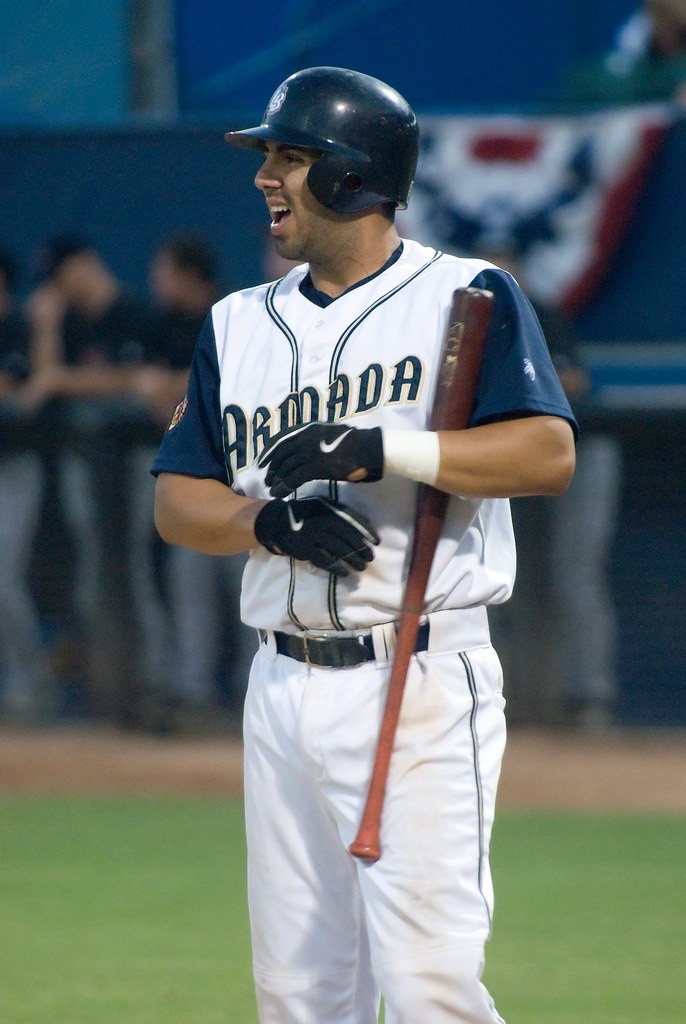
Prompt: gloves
254,498,380,579
257,420,383,498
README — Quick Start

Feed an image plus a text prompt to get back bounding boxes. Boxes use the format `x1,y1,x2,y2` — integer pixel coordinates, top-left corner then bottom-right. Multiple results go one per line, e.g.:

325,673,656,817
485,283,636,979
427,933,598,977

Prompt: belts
259,623,434,671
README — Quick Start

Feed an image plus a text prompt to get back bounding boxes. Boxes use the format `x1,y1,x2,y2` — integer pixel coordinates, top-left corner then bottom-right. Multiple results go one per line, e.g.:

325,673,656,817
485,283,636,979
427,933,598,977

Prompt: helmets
225,66,420,215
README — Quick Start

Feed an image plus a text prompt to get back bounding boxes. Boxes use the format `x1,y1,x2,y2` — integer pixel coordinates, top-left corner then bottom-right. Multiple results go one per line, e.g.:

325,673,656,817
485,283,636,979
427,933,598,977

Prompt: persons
0,233,621,735
150,65,577,1024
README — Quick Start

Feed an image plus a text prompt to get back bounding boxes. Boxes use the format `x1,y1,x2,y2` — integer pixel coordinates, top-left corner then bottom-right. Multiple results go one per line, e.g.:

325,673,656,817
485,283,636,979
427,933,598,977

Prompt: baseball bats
343,284,496,863
